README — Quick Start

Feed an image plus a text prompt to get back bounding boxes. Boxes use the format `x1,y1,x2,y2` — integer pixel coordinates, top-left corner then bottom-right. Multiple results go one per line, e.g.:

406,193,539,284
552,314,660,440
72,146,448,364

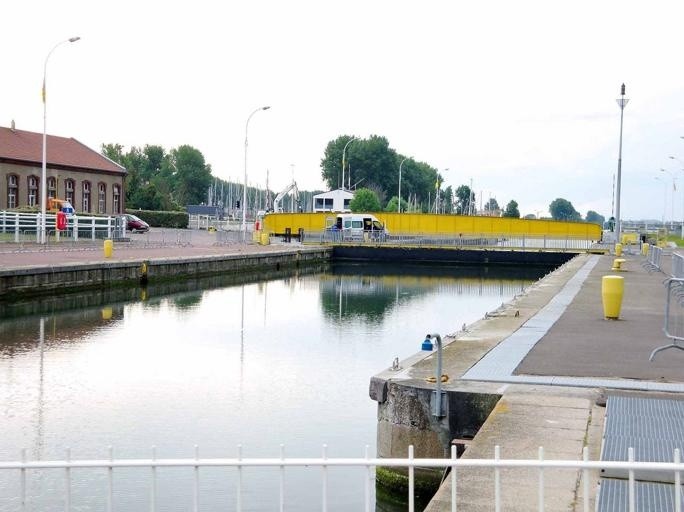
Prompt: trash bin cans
286,228,291,242
640,235,645,250
299,228,304,241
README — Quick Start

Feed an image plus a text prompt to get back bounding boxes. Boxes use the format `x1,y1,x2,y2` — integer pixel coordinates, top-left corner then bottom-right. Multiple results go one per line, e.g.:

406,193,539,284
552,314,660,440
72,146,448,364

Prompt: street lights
398,156,415,213
615,83,630,244
435,168,449,214
242,105,271,242
41,36,81,244
342,137,361,190
652,136,684,241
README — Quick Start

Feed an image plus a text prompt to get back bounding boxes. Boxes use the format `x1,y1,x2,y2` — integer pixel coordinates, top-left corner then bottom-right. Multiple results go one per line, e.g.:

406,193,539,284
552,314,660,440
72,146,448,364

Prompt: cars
120,214,150,234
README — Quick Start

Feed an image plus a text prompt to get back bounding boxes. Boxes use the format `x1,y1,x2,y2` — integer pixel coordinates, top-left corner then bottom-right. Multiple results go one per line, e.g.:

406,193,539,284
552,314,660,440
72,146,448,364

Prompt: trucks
46,198,75,213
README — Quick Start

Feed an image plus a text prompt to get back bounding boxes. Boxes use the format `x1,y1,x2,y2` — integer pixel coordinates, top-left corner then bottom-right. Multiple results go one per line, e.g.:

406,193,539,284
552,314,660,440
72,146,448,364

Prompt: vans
336,213,390,241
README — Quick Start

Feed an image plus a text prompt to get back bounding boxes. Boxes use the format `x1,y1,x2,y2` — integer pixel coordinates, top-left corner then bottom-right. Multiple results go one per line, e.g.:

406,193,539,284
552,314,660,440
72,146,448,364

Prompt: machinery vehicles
255,180,303,230
